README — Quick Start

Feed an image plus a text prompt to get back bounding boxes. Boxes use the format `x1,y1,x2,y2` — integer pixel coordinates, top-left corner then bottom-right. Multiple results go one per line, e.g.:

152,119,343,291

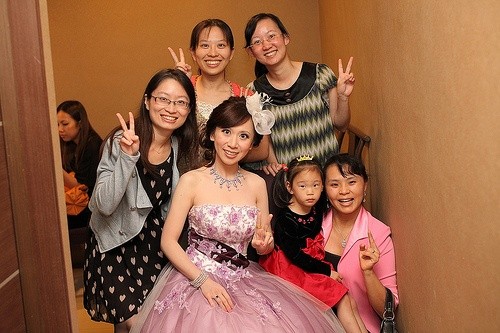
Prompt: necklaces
208,162,246,192
333,224,353,247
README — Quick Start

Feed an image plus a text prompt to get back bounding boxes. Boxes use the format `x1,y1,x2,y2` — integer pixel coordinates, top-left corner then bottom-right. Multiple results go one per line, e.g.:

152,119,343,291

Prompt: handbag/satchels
380,286,399,333
64,171,89,215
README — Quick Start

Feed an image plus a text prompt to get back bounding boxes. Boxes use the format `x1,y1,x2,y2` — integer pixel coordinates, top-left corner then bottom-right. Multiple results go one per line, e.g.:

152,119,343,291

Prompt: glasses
249,31,282,48
150,94,190,109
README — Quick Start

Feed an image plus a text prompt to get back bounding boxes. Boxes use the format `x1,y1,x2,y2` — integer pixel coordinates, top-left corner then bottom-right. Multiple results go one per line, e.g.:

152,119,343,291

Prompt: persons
169,18,268,178
82,68,198,333
319,152,400,333
126,91,349,333
257,154,371,333
56,101,104,269
245,12,356,176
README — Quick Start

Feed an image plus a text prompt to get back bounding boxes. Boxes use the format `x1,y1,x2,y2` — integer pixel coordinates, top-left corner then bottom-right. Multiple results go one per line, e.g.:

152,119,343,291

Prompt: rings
372,251,376,254
215,296,218,299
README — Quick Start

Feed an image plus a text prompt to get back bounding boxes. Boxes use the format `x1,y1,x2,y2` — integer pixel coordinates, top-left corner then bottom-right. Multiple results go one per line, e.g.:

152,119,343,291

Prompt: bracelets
191,271,207,288
